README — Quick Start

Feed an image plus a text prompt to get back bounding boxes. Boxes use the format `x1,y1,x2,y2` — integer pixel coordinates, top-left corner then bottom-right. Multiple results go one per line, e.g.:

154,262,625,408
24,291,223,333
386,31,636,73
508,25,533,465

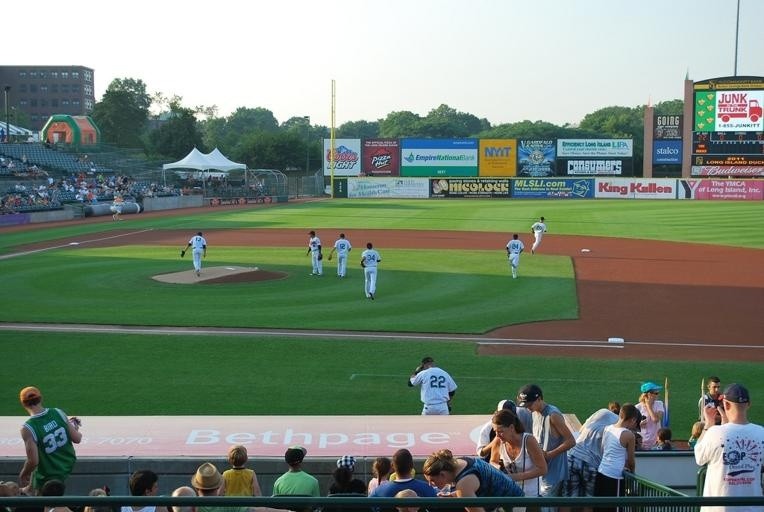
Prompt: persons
331,233,351,279
408,357,457,415
506,234,524,279
306,230,324,276
360,242,381,300
530,216,548,255
181,232,207,276
0,126,278,221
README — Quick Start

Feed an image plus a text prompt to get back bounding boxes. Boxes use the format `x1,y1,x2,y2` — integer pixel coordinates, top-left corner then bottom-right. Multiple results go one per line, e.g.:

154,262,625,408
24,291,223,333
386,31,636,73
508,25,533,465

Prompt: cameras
708,401,724,409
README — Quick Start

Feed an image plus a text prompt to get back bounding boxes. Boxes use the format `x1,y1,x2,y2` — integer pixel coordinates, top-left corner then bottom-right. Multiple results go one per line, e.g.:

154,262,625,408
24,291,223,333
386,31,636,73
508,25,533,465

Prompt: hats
497,399,516,415
515,382,543,407
639,382,663,393
335,454,357,471
717,382,749,404
421,356,434,364
190,462,224,490
19,385,41,402
284,445,307,467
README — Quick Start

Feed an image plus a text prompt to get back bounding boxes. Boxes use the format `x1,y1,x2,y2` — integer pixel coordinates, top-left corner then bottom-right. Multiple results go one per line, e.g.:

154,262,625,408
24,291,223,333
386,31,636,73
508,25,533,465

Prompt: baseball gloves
318,254,322,261
181,251,185,256
360,262,365,268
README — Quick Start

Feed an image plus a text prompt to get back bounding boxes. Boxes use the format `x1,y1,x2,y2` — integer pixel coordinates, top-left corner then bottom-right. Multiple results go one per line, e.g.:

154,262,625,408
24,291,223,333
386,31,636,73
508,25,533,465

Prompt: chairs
0,142,281,216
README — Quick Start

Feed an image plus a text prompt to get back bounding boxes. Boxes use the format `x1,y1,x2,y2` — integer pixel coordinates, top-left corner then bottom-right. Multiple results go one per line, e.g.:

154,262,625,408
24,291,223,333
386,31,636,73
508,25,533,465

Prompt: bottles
689,431,697,450
640,416,647,434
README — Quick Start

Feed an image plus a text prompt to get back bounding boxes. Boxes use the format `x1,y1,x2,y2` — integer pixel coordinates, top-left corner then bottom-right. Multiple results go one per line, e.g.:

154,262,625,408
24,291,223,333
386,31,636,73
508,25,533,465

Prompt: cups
440,484,450,496
